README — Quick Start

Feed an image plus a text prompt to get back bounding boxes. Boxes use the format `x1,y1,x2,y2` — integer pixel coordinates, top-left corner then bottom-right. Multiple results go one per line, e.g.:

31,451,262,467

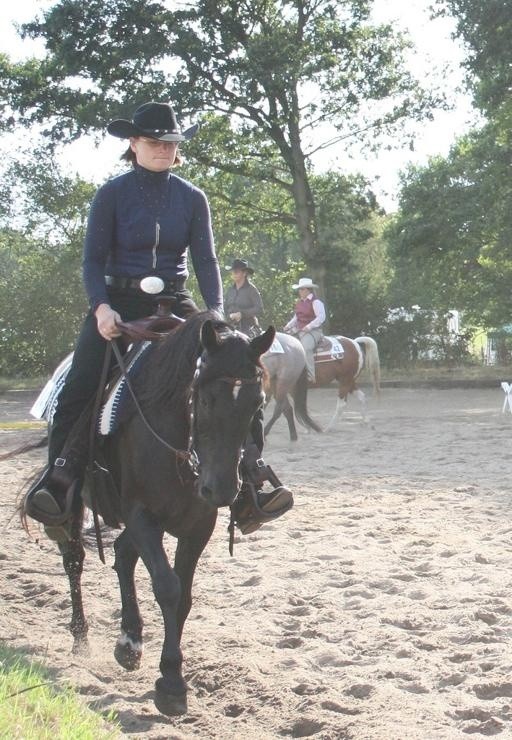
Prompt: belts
103,275,188,295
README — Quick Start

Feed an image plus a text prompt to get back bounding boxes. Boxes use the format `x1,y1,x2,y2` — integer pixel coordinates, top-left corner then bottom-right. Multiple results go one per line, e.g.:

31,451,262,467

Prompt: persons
284,278,326,384
224,259,265,341
31,104,293,541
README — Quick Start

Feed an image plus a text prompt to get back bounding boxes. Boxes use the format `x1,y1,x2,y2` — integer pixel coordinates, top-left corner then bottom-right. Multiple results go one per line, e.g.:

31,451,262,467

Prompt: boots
237,486,294,536
34,483,75,543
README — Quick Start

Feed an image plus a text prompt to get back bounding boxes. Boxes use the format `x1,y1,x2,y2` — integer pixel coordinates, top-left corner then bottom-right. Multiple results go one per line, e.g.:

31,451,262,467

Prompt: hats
291,278,319,290
107,102,199,142
225,258,255,274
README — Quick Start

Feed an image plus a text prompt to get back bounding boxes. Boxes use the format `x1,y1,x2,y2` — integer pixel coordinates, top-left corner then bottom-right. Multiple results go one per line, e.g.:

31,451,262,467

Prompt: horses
294,334,380,434
261,330,325,442
14,308,276,717
